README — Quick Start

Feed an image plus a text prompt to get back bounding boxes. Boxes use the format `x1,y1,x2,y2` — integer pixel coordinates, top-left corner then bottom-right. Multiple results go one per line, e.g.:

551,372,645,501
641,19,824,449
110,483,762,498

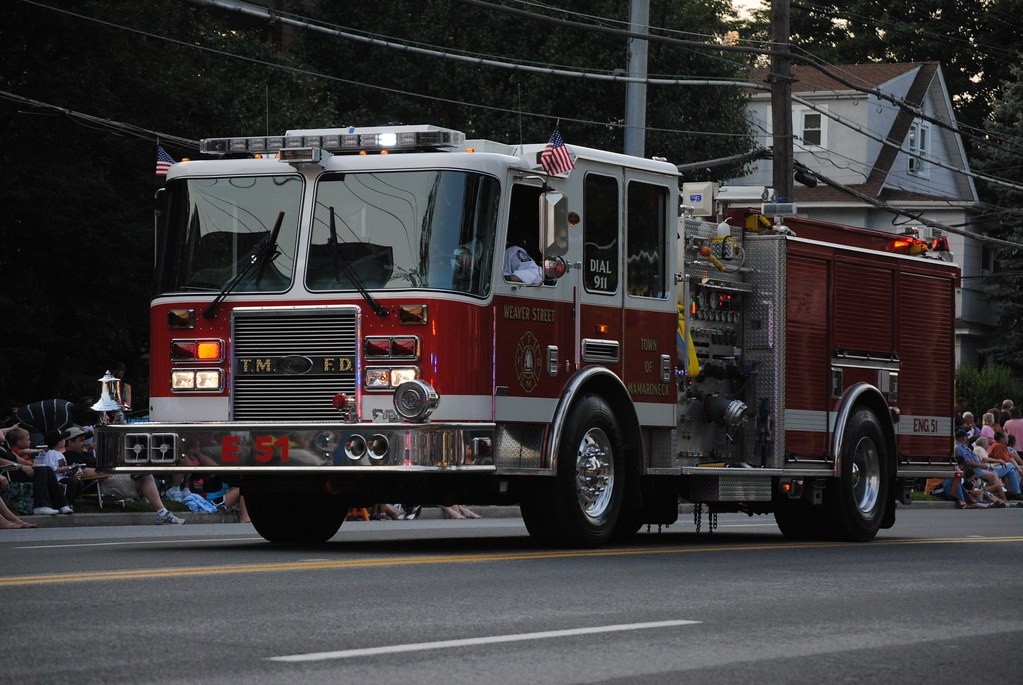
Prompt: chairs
1,465,67,514
78,475,125,510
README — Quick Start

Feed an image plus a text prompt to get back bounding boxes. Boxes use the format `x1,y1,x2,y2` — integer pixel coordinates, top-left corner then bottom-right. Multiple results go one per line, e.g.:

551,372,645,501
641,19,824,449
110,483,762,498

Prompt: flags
542,128,575,175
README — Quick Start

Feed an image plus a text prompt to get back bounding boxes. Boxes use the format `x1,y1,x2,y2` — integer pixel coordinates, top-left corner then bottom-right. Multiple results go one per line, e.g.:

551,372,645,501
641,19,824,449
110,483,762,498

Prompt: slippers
0,522,34,529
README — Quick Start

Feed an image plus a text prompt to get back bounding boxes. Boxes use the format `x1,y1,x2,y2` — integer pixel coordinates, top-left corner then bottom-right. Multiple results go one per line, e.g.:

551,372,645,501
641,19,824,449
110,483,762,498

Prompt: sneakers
33,507,58,515
156,512,186,525
59,505,73,514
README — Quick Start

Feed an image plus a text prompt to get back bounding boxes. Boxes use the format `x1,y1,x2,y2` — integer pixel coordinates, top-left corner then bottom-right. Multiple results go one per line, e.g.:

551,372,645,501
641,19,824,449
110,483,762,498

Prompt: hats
955,429,970,438
64,427,85,441
44,429,71,445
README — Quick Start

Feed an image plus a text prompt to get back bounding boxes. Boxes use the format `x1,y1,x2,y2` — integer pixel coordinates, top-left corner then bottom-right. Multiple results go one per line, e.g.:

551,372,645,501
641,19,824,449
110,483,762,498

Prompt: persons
0,362,251,529
451,225,541,293
350,505,480,522
926,399,1023,509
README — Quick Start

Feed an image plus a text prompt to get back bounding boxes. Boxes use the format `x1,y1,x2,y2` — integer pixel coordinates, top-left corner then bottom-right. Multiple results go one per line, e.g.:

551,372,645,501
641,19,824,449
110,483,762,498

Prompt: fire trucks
94,124,963,549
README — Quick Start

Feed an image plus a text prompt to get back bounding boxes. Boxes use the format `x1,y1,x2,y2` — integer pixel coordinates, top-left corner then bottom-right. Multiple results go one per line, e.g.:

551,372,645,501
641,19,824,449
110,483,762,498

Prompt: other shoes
987,499,1023,508
960,503,967,509
